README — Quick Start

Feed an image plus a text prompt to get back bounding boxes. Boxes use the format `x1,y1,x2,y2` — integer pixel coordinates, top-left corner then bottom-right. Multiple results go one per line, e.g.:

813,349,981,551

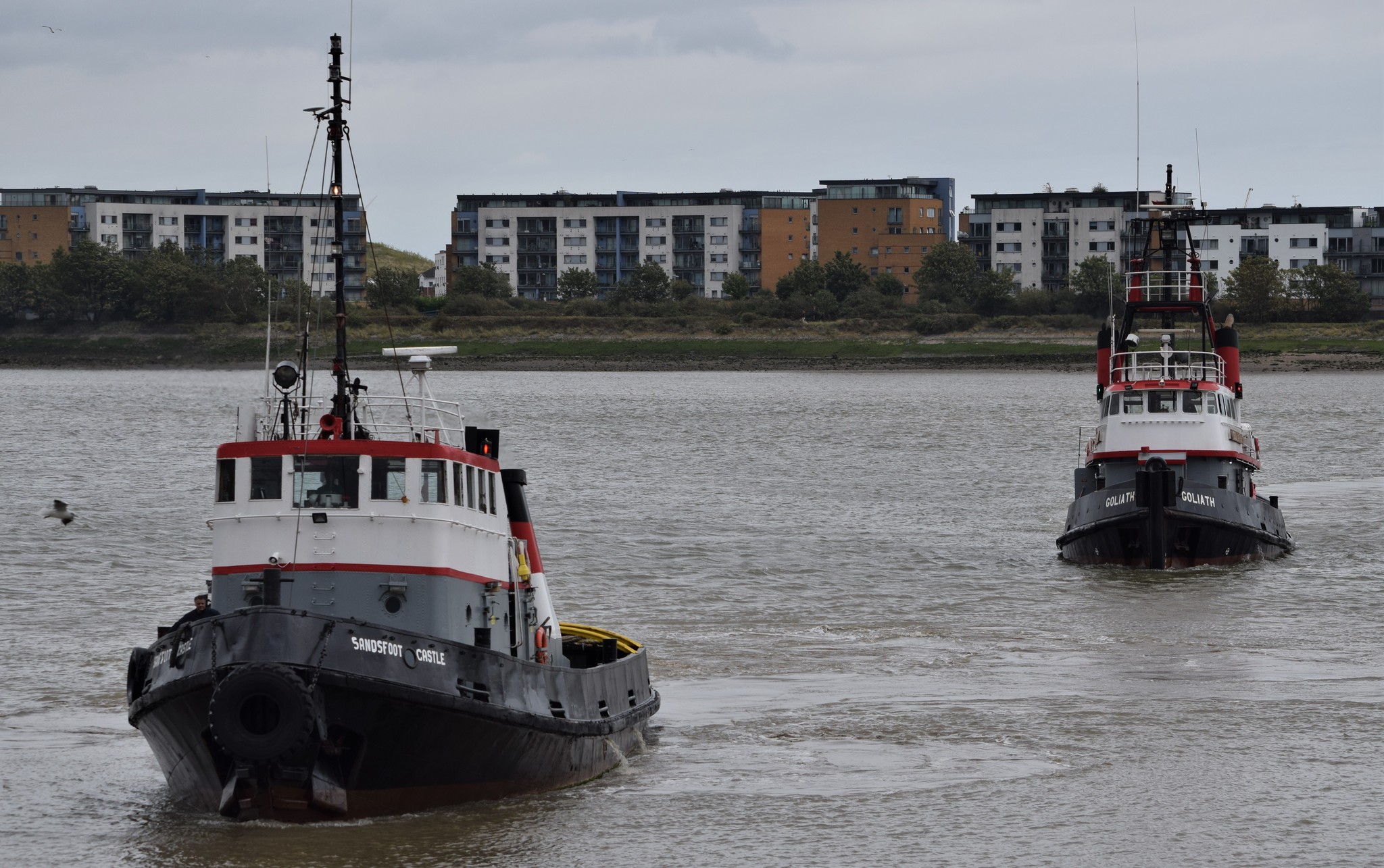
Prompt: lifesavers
208,660,316,762
1145,456,1168,472
536,627,548,664
1255,438,1261,459
1249,478,1254,498
1086,443,1092,457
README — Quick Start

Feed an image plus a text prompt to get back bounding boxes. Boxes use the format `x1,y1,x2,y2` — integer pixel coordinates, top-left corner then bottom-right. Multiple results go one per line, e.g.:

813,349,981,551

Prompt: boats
125,27,665,830
1054,144,1297,567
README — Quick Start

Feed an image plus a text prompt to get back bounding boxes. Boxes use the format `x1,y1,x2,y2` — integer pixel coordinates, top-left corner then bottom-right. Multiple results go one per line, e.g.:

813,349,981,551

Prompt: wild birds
39,499,79,525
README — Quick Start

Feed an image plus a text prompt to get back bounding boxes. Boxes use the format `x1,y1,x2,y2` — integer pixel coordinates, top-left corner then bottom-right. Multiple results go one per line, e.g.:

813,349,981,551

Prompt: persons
170,595,220,633
1183,396,1197,413
